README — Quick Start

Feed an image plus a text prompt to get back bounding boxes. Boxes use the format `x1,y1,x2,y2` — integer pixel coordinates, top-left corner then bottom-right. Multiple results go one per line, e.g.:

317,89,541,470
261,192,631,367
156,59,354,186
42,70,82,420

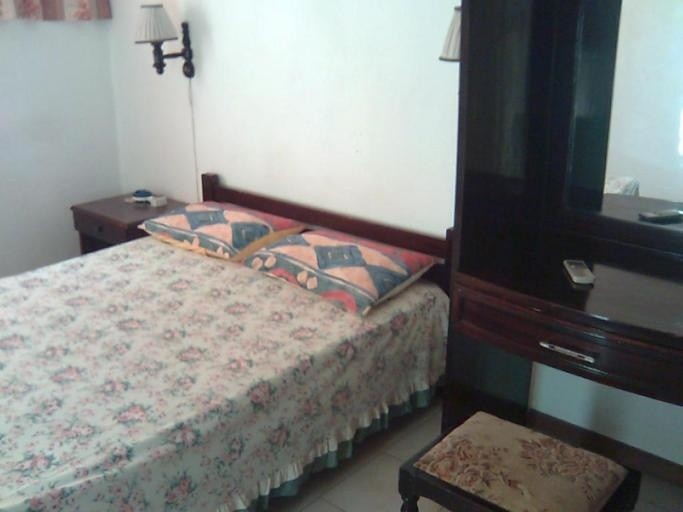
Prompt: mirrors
558,2,682,234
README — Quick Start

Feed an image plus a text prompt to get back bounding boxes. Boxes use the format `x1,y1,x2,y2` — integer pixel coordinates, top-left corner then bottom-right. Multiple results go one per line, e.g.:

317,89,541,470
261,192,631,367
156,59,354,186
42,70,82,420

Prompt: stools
399,394,642,512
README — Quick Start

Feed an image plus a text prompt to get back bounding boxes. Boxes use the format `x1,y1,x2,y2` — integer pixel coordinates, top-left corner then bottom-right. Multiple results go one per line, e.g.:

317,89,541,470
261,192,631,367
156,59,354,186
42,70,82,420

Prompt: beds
0,174,454,511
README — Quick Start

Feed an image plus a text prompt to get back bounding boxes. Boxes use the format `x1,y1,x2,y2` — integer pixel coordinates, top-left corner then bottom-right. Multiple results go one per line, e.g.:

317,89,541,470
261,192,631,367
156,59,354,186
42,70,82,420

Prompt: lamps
136,3,179,74
439,7,462,63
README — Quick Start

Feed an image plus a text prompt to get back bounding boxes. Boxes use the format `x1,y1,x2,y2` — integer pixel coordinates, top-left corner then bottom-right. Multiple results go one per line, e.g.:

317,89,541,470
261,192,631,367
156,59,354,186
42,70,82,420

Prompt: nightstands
70,191,187,255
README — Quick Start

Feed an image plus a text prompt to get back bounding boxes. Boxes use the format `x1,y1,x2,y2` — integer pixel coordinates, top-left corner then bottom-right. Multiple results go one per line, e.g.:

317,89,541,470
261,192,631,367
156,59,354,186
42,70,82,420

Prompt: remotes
639,208,683,224
563,258,596,285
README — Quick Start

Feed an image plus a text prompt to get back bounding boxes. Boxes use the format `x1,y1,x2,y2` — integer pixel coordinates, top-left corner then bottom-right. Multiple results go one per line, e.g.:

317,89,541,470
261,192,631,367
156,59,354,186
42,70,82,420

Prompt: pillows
243,228,439,318
136,199,303,265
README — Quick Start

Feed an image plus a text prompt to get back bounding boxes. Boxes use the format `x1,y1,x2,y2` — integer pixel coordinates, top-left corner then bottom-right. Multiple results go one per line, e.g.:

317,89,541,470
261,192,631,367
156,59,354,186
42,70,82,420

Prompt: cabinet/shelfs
442,0,683,470
